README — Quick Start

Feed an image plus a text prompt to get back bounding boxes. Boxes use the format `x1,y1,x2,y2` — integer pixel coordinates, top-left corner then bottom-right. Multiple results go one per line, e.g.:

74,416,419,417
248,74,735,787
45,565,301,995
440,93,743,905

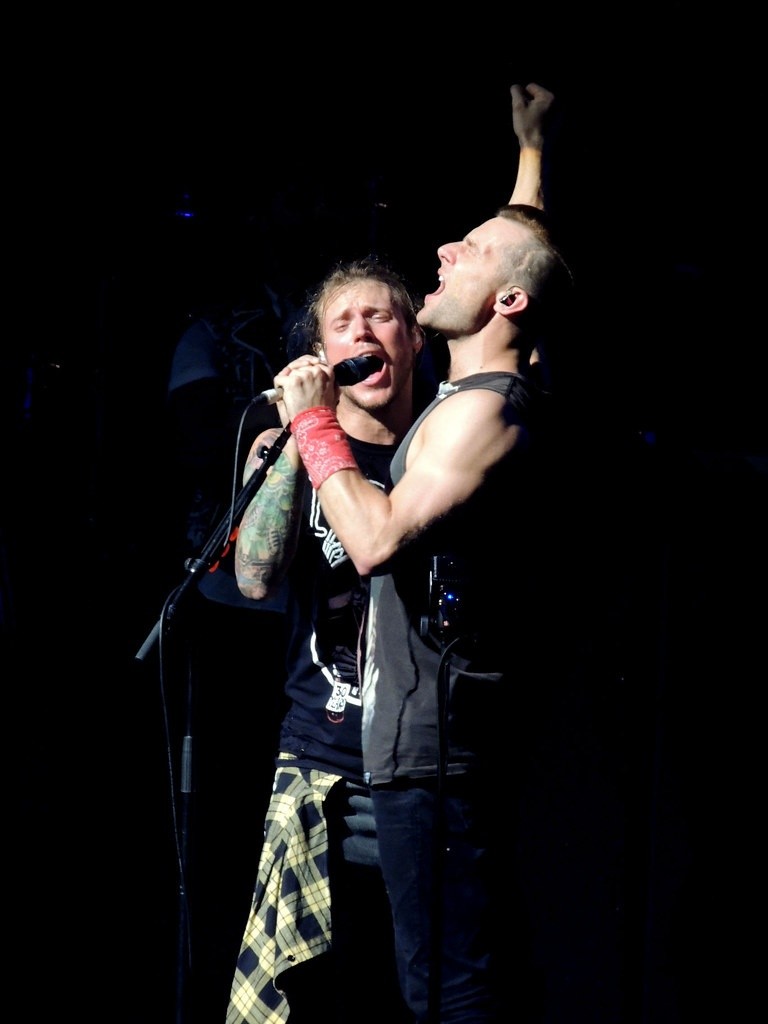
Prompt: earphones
499,293,516,306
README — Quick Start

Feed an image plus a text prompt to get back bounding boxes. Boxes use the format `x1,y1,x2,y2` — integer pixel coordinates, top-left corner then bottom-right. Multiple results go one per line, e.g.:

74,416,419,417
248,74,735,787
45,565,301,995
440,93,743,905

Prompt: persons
225,84,574,1024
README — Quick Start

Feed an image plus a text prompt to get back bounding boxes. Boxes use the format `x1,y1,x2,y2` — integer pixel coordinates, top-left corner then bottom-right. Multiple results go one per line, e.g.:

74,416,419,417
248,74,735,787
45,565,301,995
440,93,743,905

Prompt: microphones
251,357,374,407
